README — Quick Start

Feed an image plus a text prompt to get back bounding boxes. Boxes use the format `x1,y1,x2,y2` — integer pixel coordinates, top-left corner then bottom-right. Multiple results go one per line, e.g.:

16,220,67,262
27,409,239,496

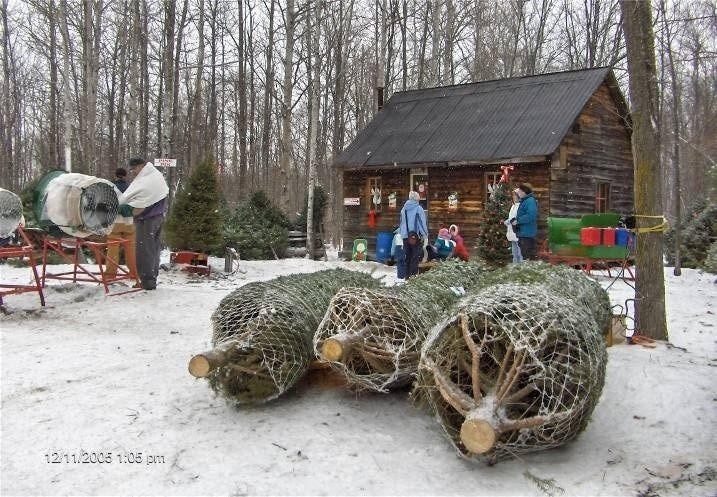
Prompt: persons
502,188,523,264
399,190,429,281
512,181,539,260
128,157,171,291
103,166,136,279
387,223,469,278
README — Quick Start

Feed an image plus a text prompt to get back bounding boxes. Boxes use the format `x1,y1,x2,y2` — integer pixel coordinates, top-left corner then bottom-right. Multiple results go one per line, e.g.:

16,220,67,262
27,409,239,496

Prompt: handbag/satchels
408,231,418,248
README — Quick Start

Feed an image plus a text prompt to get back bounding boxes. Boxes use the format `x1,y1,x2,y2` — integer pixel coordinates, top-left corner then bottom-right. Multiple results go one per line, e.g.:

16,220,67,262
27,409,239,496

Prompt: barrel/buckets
376,233,394,261
582,225,637,249
